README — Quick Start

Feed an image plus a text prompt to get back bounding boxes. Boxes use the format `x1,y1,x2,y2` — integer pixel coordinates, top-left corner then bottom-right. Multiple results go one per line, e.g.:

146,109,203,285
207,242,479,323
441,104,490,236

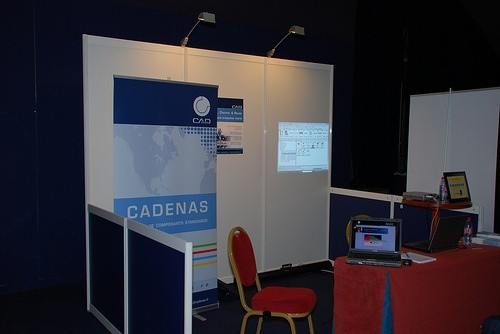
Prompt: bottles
439,177,447,202
464,217,472,248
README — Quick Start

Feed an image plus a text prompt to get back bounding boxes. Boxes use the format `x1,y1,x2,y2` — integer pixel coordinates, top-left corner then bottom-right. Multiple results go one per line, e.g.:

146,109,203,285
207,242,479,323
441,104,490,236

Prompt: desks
402,199,472,248
334,236,500,334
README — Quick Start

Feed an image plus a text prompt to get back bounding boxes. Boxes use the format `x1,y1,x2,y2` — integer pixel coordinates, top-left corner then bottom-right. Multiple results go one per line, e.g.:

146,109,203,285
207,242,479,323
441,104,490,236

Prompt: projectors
403,192,440,202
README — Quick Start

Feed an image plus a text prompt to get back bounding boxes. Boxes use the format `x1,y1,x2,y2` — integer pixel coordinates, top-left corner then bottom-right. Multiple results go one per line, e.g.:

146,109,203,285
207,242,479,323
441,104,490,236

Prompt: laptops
346,217,403,267
402,215,470,252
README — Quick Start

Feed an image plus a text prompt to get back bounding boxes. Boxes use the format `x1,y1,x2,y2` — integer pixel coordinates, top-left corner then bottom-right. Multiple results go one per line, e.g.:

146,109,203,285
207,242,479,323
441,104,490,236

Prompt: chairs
227,226,317,334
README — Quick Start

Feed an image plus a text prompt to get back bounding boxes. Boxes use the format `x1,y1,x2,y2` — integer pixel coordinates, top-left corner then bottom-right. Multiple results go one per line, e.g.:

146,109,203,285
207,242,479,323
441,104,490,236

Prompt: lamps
266,26,304,57
180,12,215,47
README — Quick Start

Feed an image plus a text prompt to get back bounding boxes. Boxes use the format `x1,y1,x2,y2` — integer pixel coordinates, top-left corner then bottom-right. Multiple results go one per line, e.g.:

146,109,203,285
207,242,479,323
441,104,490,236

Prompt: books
401,252,436,264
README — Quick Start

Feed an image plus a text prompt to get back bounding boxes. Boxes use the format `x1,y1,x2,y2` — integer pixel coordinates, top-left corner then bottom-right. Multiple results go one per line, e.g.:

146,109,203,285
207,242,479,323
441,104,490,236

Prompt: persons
217,128,226,148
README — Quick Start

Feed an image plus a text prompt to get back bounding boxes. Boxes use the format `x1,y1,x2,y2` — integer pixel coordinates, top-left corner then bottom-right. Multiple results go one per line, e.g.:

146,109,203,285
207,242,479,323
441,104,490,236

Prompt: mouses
402,259,411,266
